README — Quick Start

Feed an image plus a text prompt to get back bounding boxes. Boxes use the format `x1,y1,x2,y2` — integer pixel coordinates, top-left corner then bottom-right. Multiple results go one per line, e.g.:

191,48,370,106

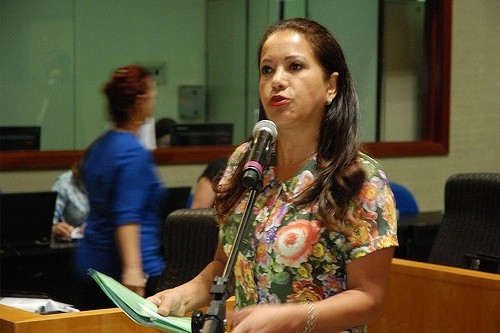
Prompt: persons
192,156,230,209
51,147,91,240
146,18,398,333
76,64,163,313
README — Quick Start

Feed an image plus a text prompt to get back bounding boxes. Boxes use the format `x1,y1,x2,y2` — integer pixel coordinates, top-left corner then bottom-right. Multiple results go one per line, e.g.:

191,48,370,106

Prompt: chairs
161,208,219,290
426,173,500,275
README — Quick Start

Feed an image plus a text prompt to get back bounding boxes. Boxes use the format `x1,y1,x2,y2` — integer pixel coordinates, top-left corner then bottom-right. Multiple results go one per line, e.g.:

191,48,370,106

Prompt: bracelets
300,301,317,333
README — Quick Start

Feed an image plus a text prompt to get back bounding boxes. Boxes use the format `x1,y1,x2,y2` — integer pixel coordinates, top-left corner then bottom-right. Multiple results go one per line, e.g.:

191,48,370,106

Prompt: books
86,268,192,333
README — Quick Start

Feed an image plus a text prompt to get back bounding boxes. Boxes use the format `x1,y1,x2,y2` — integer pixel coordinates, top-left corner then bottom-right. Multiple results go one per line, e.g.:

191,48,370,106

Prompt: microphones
242,119,278,188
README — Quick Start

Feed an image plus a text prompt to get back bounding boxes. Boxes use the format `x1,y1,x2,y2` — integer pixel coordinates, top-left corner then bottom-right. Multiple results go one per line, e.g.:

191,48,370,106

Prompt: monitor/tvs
170,123,234,147
0,126,41,151
